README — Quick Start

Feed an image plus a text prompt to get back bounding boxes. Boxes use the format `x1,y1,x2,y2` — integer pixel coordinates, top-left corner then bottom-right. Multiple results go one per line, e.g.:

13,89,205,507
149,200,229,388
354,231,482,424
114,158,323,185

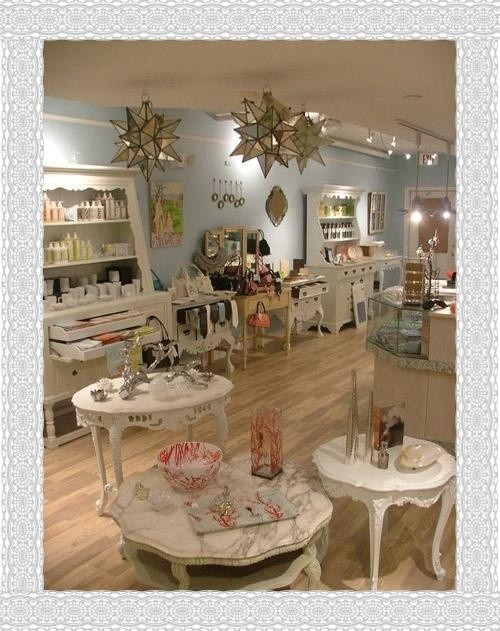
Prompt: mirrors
204,225,260,276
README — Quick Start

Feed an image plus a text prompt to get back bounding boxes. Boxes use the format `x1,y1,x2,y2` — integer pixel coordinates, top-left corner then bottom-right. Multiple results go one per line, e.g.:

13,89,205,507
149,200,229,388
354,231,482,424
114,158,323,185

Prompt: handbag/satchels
150,269,168,292
248,301,270,328
143,316,179,369
176,228,283,297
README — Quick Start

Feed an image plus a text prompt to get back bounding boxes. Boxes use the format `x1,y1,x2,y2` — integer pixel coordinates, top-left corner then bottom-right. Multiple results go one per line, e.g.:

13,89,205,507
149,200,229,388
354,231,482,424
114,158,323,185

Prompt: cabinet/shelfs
208,289,292,370
42,162,172,451
302,184,377,334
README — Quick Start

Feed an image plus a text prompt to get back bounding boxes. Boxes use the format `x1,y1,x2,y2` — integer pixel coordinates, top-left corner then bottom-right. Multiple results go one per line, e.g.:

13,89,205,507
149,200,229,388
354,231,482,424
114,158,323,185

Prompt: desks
70,372,235,518
376,256,403,313
312,432,457,591
171,293,238,376
281,275,328,350
108,457,331,592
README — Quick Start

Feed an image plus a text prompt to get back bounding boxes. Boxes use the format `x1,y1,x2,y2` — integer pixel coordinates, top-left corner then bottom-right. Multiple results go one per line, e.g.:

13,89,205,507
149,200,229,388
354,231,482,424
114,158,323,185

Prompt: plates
348,247,357,258
398,441,442,470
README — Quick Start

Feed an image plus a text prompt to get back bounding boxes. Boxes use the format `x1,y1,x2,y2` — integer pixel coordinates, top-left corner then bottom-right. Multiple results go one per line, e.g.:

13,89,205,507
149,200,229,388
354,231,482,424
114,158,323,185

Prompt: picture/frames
369,192,387,234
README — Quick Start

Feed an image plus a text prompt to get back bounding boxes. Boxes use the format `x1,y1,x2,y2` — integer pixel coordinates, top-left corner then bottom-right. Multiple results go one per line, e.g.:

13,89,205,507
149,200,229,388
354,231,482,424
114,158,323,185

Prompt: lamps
366,128,412,160
432,140,457,221
397,132,431,223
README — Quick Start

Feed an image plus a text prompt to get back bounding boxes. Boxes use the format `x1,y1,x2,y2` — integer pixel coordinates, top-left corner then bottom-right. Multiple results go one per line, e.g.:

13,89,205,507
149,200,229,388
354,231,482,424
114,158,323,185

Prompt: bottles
377,440,389,470
